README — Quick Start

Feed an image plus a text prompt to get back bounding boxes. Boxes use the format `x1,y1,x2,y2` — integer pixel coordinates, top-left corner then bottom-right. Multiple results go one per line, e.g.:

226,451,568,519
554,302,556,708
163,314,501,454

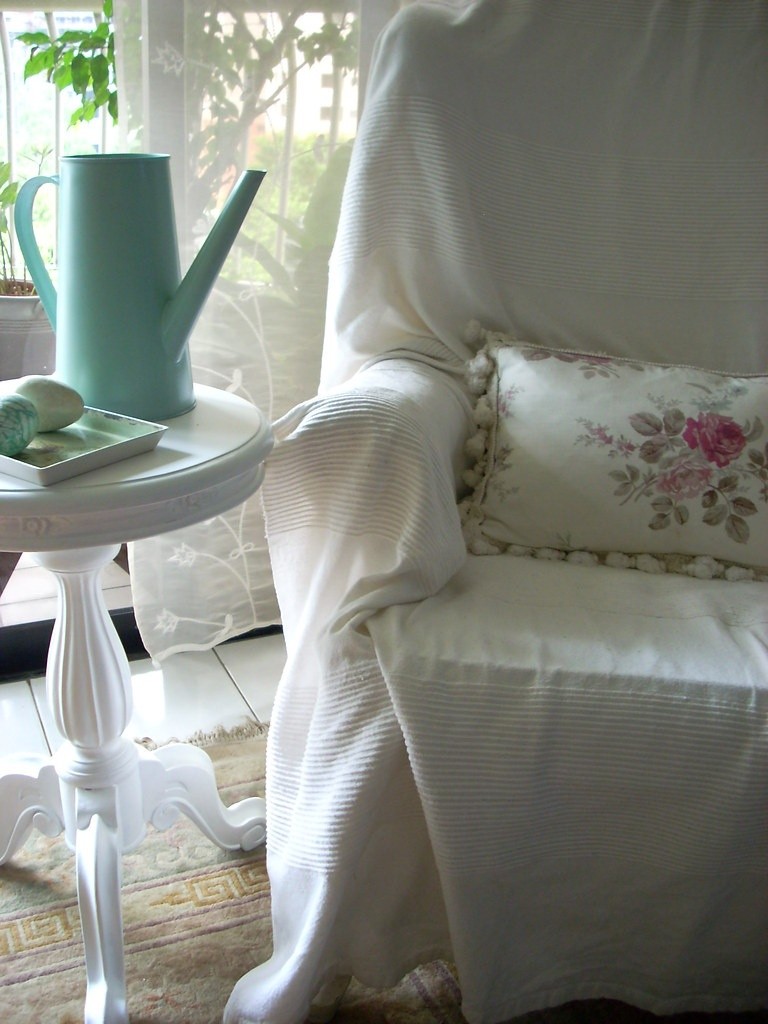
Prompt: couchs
221,1,767,1024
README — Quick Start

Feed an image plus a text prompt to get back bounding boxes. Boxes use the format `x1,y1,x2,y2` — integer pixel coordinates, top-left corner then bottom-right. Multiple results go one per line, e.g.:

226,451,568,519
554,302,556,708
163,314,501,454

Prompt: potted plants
0,141,56,322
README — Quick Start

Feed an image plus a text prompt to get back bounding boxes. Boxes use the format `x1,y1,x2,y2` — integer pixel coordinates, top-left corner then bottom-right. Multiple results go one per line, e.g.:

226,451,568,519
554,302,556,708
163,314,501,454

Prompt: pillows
458,320,768,584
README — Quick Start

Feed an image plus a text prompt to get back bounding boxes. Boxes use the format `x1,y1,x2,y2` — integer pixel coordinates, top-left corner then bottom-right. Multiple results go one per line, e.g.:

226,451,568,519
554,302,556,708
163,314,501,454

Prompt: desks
0,374,278,1024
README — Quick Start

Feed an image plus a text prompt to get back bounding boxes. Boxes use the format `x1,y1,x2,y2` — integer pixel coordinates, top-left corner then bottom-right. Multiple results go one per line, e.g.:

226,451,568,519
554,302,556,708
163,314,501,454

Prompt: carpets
0,716,462,1024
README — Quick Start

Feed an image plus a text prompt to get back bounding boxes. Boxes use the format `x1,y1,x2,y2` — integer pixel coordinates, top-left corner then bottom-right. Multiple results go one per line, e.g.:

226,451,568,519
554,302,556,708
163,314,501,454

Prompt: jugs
13,153,268,422
